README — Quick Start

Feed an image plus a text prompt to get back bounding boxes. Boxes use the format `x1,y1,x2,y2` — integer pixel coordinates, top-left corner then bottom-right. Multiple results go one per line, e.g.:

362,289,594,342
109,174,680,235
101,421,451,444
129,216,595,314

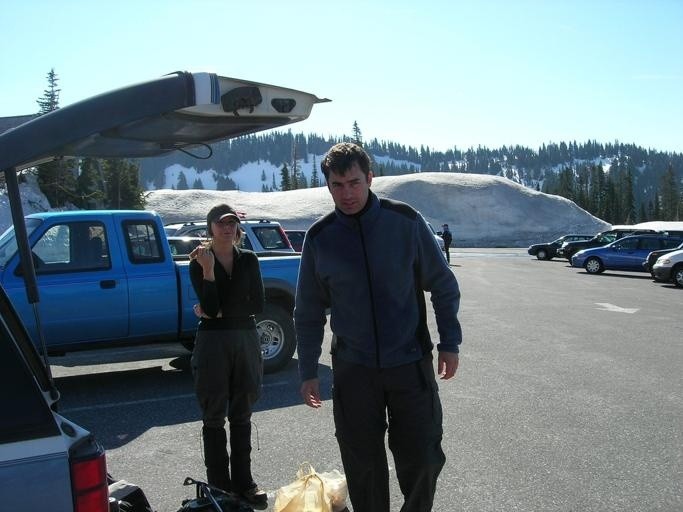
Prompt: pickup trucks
0,210,301,373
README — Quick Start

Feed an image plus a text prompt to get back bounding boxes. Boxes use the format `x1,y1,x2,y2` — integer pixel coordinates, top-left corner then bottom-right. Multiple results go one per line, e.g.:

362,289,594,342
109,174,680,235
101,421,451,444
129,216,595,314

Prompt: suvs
0,69,332,512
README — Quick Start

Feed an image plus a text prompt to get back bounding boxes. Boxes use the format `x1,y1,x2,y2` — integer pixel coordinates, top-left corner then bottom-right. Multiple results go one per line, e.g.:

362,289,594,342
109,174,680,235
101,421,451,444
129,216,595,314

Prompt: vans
528,229,683,288
161,219,308,252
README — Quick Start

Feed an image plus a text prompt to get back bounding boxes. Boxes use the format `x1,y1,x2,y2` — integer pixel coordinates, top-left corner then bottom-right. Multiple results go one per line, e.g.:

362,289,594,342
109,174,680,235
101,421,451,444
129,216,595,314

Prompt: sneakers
237,485,267,505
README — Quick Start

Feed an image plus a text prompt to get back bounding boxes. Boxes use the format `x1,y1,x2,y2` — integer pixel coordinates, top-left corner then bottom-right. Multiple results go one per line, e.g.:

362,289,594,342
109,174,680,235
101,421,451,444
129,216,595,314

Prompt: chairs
82,236,103,269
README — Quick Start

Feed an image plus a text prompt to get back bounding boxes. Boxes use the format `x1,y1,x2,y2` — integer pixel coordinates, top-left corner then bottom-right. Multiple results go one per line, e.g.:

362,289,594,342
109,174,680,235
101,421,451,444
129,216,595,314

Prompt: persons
440,224,452,264
293,143,462,512
191,204,269,504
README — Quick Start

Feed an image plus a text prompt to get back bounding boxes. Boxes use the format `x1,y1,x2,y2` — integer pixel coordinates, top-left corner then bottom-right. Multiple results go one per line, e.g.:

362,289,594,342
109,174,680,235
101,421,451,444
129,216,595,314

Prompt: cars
429,221,447,261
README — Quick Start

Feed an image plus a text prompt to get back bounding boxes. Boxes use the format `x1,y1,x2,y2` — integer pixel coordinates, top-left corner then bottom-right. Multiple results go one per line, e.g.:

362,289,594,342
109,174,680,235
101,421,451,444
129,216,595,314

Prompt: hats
207,204,240,225
441,224,448,227
